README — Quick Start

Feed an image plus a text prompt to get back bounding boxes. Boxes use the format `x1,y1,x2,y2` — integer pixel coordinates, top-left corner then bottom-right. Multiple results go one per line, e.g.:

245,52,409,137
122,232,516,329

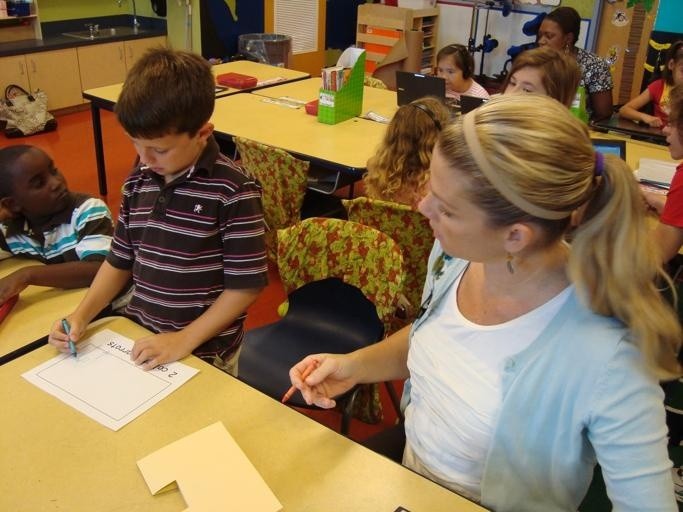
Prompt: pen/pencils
280,360,320,402
60,318,78,358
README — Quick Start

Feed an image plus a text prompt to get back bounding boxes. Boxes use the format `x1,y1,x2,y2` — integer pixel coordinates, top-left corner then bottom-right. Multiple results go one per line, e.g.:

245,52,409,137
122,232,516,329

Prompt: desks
600,115,671,141
82,59,311,196
3,312,490,511
584,131,682,258
1,246,114,370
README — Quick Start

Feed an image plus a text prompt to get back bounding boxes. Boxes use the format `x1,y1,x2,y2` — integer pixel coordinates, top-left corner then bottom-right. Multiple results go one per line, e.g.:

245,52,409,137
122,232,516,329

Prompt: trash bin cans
238,32,292,70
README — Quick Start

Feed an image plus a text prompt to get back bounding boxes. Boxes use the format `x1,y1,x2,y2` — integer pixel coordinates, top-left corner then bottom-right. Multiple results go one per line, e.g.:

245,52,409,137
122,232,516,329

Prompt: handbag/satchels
0,85,57,138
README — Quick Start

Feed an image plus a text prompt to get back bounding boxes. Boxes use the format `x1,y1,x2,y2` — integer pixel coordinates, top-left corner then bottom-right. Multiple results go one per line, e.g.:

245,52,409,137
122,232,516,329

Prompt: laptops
591,139,626,162
395,71,446,107
460,95,488,115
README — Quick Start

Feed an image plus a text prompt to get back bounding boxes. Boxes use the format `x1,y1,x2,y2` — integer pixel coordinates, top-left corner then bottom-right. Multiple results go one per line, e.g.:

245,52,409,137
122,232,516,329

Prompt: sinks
61,27,147,40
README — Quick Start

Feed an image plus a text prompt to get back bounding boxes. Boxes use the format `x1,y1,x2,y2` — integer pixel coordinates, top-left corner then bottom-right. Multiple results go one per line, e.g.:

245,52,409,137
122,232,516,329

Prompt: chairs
232,136,346,266
237,216,409,437
346,196,439,314
201,76,462,219
361,74,389,94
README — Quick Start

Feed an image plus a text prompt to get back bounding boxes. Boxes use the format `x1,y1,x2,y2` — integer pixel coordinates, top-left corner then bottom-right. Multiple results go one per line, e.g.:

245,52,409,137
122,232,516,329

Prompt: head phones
450,45,473,78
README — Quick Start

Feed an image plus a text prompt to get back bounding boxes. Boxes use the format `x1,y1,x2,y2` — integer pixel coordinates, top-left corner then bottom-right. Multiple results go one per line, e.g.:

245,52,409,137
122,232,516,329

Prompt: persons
47,43,269,382
534,7,611,121
617,39,682,127
0,140,123,305
648,86,683,260
367,97,456,209
434,43,491,108
501,49,580,114
288,97,682,510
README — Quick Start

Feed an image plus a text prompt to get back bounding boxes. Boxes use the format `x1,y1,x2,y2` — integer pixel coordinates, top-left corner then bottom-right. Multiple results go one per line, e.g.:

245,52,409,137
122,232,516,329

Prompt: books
320,65,350,89
629,155,677,195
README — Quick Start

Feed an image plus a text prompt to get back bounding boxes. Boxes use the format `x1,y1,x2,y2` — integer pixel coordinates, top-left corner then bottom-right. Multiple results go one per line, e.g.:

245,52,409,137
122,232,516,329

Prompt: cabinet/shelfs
77,36,167,113
355,3,441,90
0,47,83,130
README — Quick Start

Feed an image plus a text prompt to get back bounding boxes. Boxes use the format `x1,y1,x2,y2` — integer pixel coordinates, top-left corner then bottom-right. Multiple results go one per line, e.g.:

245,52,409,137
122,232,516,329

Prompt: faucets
118,0,140,28
